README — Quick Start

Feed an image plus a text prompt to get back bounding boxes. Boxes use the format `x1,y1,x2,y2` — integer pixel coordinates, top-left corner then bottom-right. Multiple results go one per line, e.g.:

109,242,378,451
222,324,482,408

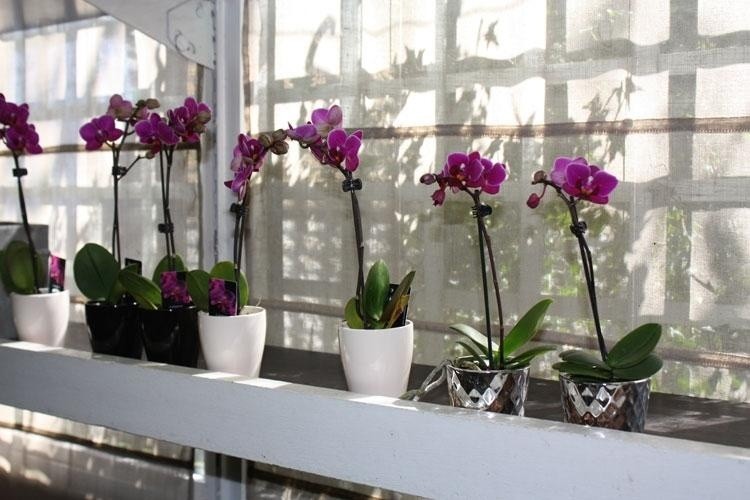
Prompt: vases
12,292,269,379
